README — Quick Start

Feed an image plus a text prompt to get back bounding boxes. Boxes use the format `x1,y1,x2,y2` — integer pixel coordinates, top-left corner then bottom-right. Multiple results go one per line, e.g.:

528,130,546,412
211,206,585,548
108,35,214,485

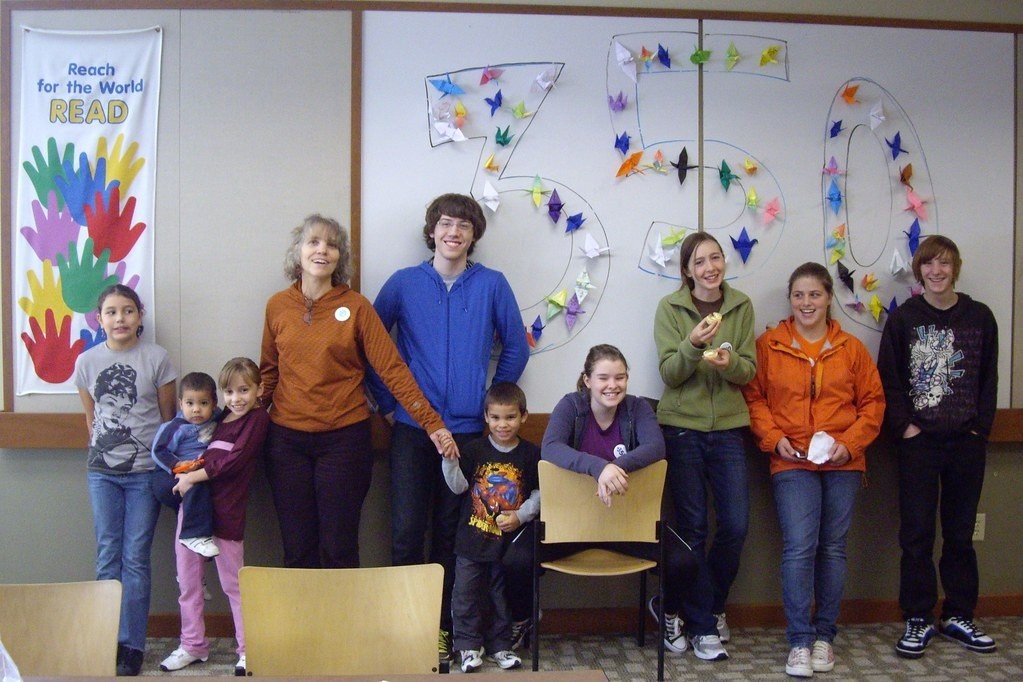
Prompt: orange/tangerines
707,312,722,324
704,348,718,360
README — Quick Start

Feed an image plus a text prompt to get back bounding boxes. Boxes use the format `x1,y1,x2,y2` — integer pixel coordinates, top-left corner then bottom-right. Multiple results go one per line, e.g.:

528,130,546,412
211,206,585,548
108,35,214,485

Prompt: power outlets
972,512,986,541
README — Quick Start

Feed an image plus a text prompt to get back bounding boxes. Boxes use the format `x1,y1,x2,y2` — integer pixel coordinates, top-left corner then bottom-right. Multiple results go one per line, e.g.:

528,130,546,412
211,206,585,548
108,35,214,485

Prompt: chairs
530,457,668,682
238,563,444,677
0,580,123,675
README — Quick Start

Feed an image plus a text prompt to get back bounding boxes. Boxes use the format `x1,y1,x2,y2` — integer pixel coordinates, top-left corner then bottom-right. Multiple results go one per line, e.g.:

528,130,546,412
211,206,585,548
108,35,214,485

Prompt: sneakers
713,613,730,644
690,635,730,660
459,645,485,673
178,535,220,558
895,616,938,659
485,649,522,670
438,628,452,660
159,645,210,671
118,645,144,676
785,642,813,677
508,606,542,650
937,615,998,653
810,640,836,672
648,595,688,653
233,655,246,677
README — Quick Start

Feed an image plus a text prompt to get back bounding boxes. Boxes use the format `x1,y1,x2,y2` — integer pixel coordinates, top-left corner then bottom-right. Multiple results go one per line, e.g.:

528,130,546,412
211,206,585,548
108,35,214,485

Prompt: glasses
436,218,473,232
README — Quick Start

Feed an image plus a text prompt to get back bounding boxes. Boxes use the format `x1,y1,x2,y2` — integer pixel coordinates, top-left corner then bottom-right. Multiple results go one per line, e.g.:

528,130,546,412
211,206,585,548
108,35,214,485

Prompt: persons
76,283,178,678
150,355,271,676
742,260,886,677
501,231,755,682
877,234,999,659
259,194,542,675
